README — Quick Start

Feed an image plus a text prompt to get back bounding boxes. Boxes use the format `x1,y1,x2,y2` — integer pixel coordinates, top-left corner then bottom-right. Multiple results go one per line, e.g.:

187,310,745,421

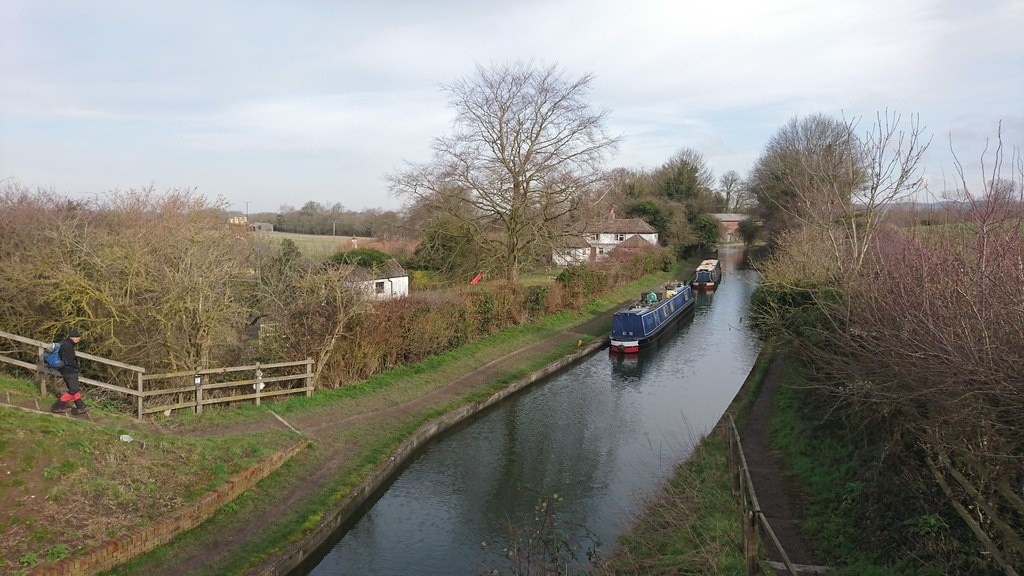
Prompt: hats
69,328,80,338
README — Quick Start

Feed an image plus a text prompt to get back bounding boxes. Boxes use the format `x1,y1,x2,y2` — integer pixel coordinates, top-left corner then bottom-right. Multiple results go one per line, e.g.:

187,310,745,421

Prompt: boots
72,392,90,415
50,391,74,413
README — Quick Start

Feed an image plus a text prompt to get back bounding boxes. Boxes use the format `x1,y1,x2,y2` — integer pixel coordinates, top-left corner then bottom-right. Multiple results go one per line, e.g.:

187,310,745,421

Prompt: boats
693,258,724,296
608,279,695,355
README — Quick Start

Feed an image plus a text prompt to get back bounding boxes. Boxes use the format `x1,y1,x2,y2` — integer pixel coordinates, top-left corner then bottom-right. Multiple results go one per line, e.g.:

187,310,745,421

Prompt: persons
51,329,92,414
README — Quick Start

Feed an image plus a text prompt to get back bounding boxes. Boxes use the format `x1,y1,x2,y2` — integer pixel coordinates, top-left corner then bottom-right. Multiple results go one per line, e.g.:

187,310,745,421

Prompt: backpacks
44,343,66,368
